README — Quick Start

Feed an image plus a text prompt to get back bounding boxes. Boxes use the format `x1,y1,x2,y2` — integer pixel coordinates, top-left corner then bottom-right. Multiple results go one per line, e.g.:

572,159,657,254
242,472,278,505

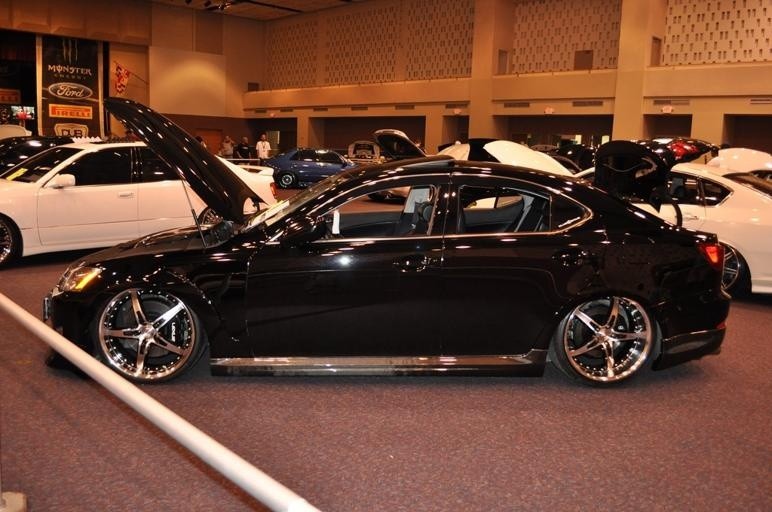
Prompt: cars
1,136,281,269
44,154,731,388
260,146,361,191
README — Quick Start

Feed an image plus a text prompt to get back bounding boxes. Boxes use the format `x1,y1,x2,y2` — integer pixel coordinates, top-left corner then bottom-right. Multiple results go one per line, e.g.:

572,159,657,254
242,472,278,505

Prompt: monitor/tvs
10,104,36,121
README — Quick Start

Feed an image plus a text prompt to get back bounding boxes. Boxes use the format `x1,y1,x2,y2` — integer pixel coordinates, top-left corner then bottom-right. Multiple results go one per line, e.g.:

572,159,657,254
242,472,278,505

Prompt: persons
193,136,208,149
219,132,272,171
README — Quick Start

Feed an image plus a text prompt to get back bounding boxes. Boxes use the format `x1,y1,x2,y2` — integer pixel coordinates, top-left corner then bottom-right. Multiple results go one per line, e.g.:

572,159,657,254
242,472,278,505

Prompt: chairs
404,202,432,237
672,185,698,202
